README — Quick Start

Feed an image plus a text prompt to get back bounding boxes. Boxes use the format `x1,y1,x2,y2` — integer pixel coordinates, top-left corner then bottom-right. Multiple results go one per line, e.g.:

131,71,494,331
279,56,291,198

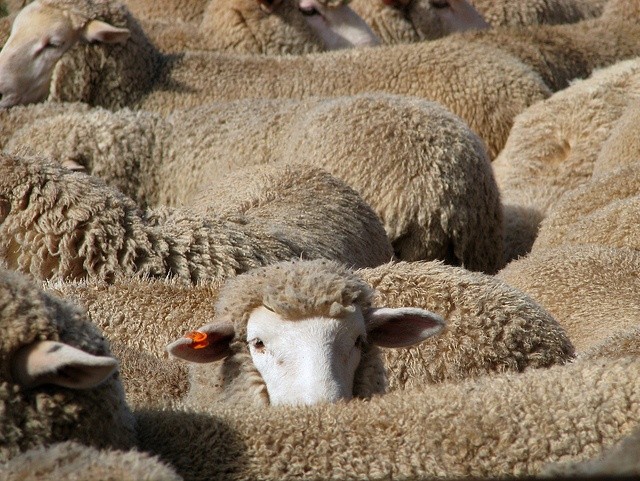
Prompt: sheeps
1,152,395,284
1,0,553,160
166,258,578,405
494,58,640,259
502,101,639,359
469,1,603,30
0,269,640,480
343,0,492,45
448,0,640,91
0,0,379,53
1,93,506,274
